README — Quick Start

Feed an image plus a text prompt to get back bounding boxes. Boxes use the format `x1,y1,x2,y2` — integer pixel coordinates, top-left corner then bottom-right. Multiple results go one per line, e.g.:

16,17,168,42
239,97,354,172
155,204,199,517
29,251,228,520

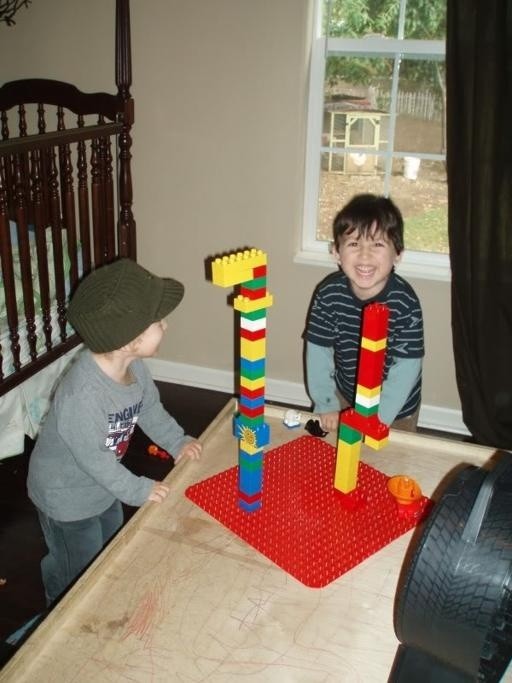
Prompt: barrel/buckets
403,157,420,179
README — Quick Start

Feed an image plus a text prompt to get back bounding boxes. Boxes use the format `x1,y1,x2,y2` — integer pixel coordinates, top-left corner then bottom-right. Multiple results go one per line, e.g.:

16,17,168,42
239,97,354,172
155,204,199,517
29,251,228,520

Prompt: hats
66,257,184,354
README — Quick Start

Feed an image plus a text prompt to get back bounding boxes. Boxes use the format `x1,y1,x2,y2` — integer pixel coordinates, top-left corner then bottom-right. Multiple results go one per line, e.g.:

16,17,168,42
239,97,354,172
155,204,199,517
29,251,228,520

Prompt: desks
0,391,512,682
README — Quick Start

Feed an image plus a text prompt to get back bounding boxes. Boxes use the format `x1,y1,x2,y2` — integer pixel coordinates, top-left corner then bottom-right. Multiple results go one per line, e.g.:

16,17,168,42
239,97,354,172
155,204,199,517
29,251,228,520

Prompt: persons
24,256,204,609
301,188,425,433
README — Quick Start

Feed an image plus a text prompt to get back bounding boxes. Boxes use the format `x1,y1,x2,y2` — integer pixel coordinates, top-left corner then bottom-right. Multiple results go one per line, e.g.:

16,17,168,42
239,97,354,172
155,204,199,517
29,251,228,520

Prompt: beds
0,0,163,516
